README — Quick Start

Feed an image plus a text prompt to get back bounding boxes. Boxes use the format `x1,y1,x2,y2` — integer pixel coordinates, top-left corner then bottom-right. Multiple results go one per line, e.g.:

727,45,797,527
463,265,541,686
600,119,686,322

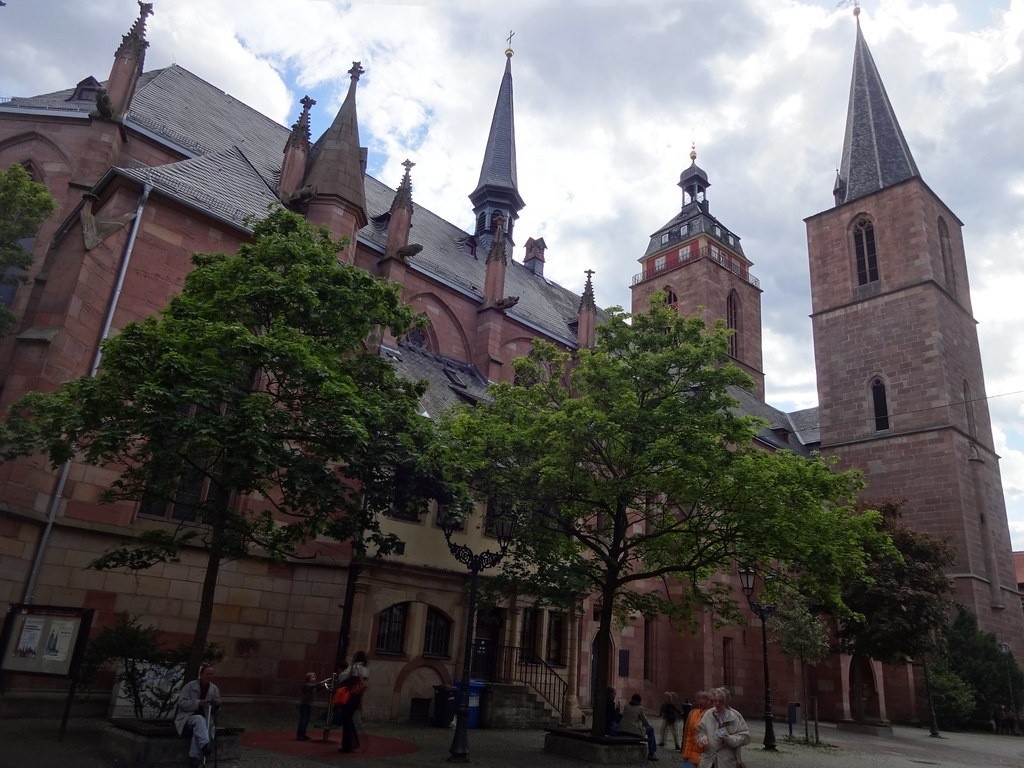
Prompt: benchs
108,717,246,734
544,728,650,742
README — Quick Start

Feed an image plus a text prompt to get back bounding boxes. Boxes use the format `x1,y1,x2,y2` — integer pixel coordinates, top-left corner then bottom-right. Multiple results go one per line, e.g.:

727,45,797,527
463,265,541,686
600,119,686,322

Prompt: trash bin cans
433,683,458,729
460,679,493,730
409,696,432,725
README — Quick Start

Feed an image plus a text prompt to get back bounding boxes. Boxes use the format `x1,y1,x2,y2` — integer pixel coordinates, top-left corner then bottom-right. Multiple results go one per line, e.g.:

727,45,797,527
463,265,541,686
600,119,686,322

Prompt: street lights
737,566,781,752
998,641,1019,735
439,495,519,763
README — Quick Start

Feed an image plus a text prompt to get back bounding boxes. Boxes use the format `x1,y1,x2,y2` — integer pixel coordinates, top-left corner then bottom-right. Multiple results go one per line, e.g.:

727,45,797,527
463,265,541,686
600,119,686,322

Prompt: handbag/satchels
342,662,369,695
332,663,354,705
736,761,746,768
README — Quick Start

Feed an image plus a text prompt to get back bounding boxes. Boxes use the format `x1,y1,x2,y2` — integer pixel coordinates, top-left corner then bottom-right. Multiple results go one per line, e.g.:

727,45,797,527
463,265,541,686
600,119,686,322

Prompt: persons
681,692,715,768
339,650,367,753
606,686,623,735
173,665,222,768
656,692,683,750
696,688,750,768
619,693,660,762
296,672,329,741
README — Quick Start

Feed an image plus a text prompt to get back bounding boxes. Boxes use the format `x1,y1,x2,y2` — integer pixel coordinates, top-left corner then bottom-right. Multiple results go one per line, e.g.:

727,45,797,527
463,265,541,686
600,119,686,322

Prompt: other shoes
190,757,206,768
337,742,361,753
296,736,311,742
202,743,213,757
658,742,664,746
647,753,659,761
675,744,681,751
